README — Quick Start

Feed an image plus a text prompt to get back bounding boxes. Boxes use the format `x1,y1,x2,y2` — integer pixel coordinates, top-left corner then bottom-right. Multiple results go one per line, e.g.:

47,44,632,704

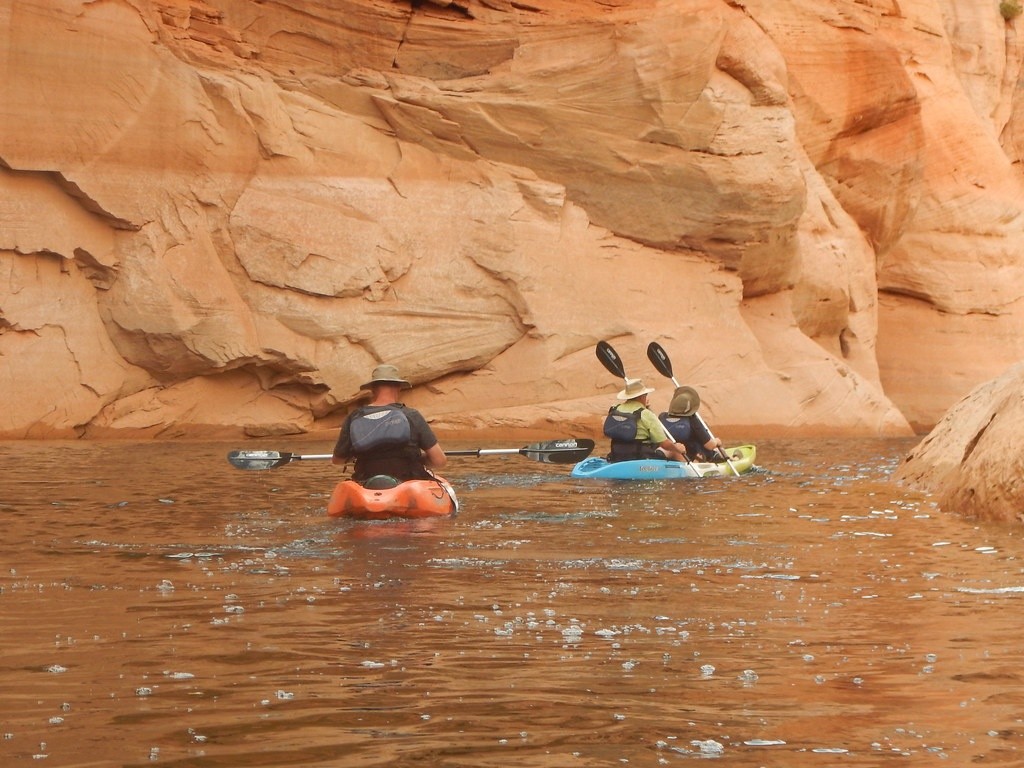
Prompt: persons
658,386,739,463
604,379,707,463
333,364,447,486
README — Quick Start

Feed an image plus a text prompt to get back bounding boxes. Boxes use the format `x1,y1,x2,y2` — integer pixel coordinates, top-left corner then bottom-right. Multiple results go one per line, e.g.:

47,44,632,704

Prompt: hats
360,365,413,390
617,379,655,399
668,386,700,417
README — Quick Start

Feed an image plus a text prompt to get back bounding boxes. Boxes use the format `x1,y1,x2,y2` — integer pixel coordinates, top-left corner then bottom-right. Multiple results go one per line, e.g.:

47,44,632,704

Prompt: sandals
692,452,707,463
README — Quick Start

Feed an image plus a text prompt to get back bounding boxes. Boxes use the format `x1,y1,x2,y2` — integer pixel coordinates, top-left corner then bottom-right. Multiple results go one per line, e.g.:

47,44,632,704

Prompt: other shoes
733,449,743,461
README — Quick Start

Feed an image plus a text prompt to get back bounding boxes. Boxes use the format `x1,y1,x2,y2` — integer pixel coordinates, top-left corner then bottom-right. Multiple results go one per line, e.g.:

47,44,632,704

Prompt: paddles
647,342,742,480
595,339,704,478
227,438,595,470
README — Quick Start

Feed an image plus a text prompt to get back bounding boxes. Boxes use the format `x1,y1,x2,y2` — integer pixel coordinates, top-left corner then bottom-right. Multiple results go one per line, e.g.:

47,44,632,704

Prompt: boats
327,469,458,518
572,445,757,480
